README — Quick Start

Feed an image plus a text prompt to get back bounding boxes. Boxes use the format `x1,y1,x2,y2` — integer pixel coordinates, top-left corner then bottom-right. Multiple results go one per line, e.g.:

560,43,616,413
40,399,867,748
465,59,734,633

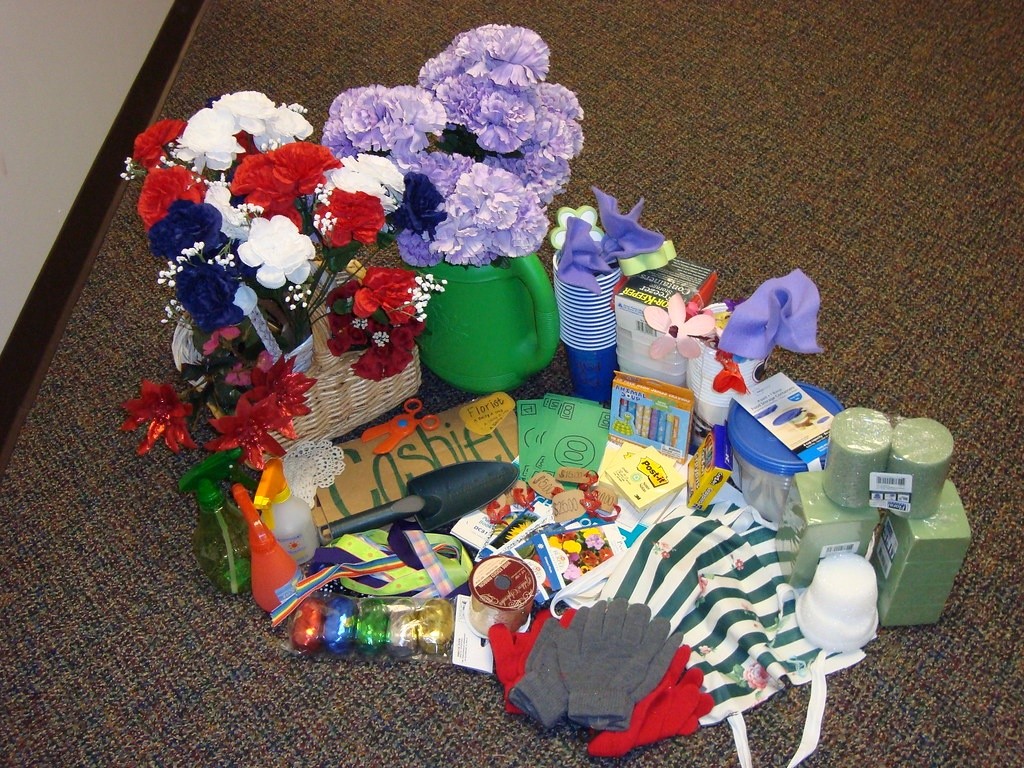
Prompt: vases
415,252,563,394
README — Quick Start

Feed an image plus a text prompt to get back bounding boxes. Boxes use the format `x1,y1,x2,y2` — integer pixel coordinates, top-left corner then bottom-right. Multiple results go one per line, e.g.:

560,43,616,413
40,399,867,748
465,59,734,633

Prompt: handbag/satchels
594,501,867,768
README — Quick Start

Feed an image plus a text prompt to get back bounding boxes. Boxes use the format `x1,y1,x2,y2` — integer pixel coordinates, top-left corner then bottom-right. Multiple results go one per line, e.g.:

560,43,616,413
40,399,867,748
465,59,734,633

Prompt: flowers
643,292,717,360
120,91,447,472
319,22,587,267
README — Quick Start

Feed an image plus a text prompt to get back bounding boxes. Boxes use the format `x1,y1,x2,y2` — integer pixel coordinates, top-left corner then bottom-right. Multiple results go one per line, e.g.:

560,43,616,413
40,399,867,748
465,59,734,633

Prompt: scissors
361,398,440,456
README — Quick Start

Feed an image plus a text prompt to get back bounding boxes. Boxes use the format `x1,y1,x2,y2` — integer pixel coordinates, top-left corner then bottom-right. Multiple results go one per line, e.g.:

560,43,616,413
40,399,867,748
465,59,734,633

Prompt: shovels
316,460,519,547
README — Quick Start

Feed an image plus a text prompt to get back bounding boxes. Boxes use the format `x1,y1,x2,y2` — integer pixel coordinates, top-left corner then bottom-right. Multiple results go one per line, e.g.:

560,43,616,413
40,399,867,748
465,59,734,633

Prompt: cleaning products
232,459,320,616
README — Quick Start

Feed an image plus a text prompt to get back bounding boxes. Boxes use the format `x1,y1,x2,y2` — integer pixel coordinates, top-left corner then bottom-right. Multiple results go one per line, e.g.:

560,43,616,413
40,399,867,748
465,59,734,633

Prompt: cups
552,248,624,405
684,302,771,457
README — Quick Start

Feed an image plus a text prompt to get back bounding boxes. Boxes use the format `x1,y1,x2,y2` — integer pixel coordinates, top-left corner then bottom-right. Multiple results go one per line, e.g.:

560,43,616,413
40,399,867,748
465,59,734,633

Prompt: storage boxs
687,424,733,511
613,257,718,388
726,383,845,523
610,371,696,464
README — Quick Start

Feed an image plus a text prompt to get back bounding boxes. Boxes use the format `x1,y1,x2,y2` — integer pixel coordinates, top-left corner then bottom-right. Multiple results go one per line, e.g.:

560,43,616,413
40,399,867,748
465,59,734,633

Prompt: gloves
488,598,714,758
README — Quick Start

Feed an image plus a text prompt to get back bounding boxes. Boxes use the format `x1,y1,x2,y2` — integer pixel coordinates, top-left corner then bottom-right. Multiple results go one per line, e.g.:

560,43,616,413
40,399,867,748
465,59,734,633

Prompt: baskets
172,260,422,470
